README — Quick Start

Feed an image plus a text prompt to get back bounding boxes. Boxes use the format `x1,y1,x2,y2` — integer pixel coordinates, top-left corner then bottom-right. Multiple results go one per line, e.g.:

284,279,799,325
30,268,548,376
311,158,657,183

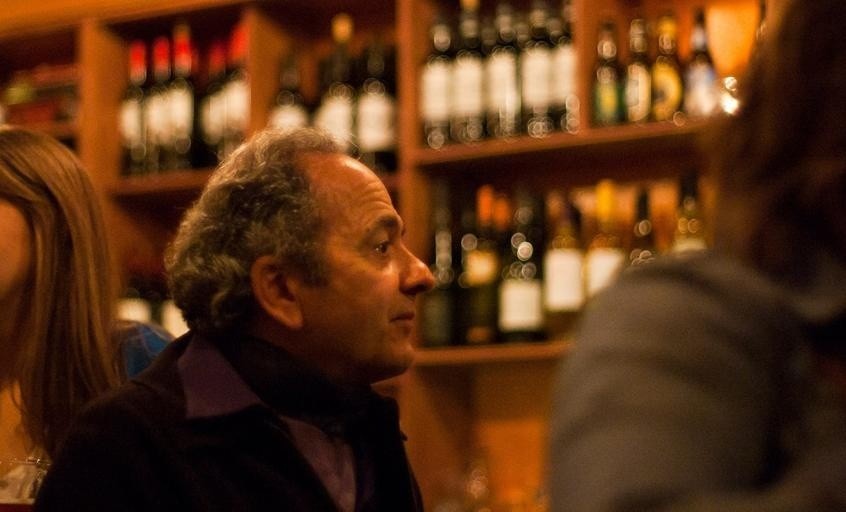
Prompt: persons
29,127,436,512
0,125,178,512
543,0,845,512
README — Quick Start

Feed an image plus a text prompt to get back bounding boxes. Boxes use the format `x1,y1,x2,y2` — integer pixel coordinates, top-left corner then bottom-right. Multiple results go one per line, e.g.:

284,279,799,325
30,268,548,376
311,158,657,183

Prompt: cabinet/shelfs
113,2,408,192
1,1,113,192
409,0,777,364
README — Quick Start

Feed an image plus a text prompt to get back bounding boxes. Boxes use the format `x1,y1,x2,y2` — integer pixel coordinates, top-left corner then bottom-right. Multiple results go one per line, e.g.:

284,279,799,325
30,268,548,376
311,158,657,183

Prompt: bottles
422,162,702,346
119,251,188,337
418,0,577,168
272,13,392,171
117,12,253,179
589,4,717,127
